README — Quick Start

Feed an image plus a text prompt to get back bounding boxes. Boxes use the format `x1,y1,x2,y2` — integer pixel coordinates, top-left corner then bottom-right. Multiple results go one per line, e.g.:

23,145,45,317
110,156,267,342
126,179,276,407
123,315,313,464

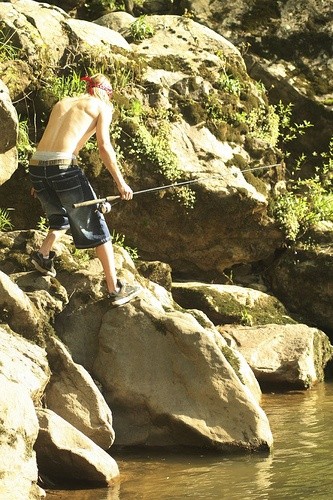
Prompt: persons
26,73,141,306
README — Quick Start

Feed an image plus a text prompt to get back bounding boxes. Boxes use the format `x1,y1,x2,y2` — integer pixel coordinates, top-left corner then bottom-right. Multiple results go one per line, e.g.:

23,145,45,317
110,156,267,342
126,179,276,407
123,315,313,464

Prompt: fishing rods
71,162,285,216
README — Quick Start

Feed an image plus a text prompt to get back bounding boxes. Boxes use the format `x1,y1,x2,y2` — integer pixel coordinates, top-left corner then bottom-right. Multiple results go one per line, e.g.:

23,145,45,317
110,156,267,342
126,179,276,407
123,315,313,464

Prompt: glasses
86,81,113,98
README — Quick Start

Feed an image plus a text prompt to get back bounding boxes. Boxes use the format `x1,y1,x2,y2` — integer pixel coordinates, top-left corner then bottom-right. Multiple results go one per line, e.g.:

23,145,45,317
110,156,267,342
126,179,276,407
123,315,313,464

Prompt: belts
29,159,82,167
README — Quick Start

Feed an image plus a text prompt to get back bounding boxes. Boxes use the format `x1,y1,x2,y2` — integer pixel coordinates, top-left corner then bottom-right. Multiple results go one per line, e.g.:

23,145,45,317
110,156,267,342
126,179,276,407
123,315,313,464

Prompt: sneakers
108,278,142,305
26,250,57,277
